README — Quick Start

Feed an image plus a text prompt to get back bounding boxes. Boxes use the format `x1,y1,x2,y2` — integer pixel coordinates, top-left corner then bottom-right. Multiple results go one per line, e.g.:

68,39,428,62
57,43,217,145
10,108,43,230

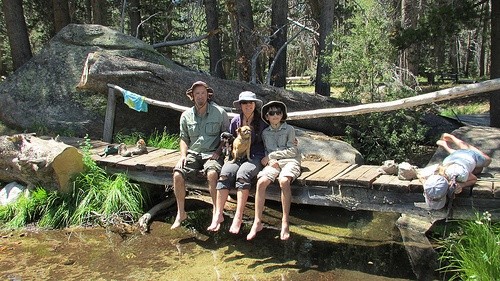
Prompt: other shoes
116,143,131,156
131,139,148,155
104,143,120,154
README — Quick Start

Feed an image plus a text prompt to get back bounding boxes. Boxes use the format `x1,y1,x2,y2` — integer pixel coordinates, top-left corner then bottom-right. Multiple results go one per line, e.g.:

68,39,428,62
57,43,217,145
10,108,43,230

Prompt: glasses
240,101,255,104
265,109,284,116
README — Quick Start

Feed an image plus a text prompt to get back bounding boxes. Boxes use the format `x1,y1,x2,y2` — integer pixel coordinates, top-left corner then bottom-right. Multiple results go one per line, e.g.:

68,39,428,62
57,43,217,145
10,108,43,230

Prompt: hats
261,101,288,125
233,91,263,110
423,175,449,209
186,81,214,101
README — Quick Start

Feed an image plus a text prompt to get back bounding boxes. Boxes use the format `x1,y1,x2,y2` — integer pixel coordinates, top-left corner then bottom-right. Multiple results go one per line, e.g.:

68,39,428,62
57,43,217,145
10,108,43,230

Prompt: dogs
228,125,253,164
220,132,235,161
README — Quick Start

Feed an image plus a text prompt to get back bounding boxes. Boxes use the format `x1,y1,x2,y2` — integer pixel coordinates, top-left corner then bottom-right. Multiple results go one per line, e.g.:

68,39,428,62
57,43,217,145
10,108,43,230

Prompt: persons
247,101,303,240
424,133,491,211
171,81,229,232
207,91,298,234
